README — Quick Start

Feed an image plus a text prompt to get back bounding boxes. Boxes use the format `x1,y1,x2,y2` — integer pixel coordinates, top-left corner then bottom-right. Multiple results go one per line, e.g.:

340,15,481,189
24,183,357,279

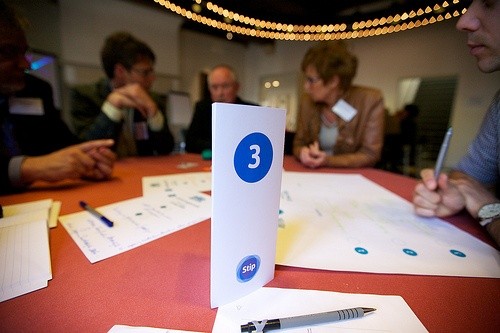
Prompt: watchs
476,202,500,233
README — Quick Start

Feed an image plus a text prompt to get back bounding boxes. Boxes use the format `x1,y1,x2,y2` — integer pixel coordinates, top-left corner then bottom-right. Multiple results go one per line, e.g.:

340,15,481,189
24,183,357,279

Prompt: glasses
132,67,153,78
305,75,321,84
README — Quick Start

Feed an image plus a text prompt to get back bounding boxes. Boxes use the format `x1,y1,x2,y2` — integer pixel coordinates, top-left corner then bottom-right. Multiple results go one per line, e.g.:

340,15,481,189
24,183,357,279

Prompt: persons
0,6,117,191
71,29,175,164
379,105,418,173
288,40,388,169
413,0,500,250
184,64,267,157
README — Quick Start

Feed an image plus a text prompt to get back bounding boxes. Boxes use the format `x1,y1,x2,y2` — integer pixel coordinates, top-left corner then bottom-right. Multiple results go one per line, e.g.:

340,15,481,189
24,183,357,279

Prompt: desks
0,155,500,333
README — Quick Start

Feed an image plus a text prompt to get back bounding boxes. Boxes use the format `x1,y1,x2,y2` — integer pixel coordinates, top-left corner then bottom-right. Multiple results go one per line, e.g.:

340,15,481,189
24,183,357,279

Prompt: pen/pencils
80,201,113,226
241,308,376,333
434,128,453,180
